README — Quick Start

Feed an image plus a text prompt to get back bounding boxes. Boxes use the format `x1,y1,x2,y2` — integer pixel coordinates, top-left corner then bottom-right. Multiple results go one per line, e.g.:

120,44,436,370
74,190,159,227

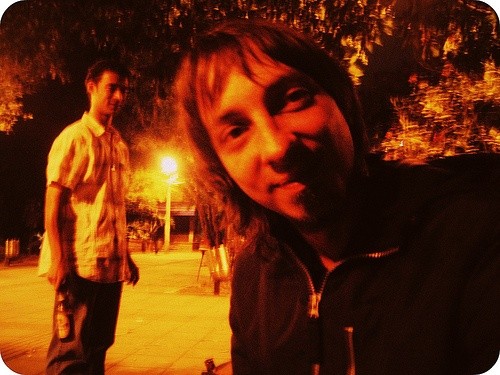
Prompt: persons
173,20,500,374
36,62,139,375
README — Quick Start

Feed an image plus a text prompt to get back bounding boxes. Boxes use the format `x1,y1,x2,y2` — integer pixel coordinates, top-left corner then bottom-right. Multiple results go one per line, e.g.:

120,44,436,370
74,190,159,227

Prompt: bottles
204,359,216,372
56,278,75,343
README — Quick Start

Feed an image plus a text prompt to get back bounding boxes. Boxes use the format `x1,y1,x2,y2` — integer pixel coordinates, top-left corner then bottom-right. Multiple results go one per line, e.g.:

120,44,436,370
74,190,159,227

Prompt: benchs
196,248,209,281
205,247,232,296
